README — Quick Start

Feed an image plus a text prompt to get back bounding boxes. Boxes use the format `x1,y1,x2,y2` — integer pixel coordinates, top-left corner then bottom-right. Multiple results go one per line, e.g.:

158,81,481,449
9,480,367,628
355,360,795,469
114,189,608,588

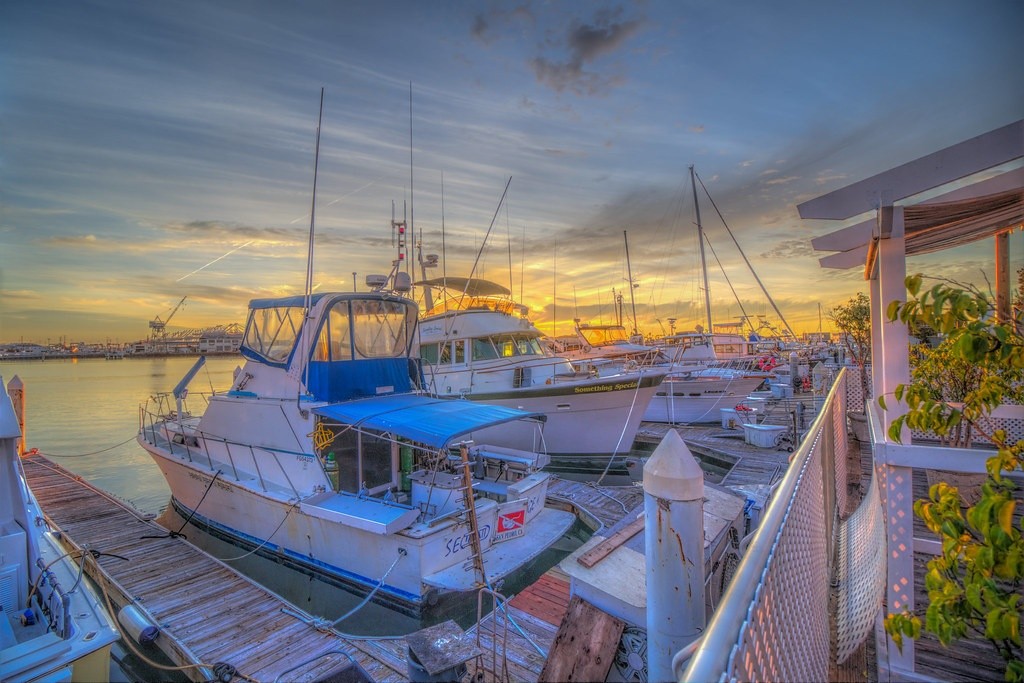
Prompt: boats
353,83,828,475
0,375,121,683
137,87,577,607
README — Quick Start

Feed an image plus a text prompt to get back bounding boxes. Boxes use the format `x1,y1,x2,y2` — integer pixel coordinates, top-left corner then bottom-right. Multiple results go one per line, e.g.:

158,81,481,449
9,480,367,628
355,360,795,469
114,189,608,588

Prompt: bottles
325,452,339,492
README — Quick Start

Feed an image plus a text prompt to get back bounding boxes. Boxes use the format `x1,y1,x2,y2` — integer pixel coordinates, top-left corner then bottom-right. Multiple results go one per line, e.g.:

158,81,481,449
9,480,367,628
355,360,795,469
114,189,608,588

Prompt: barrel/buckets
406,645,468,683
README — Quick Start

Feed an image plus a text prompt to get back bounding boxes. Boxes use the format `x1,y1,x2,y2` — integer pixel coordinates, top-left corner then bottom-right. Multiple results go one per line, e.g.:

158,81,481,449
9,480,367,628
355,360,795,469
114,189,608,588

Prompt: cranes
149,295,187,339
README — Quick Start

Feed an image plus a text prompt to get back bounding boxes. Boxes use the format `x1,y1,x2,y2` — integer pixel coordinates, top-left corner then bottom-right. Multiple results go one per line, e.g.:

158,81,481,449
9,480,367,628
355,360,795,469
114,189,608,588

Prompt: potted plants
908,343,1000,510
824,291,873,443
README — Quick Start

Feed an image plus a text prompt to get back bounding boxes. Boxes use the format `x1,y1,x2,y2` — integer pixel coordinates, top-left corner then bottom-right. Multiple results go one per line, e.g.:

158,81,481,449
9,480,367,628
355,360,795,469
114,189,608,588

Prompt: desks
470,479,510,501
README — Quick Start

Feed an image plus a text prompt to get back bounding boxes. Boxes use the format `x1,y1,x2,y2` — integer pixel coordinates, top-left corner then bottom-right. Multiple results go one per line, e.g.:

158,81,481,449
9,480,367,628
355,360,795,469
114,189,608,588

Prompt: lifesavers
794,381,801,387
802,376,810,388
793,375,801,383
758,355,774,371
583,345,591,352
